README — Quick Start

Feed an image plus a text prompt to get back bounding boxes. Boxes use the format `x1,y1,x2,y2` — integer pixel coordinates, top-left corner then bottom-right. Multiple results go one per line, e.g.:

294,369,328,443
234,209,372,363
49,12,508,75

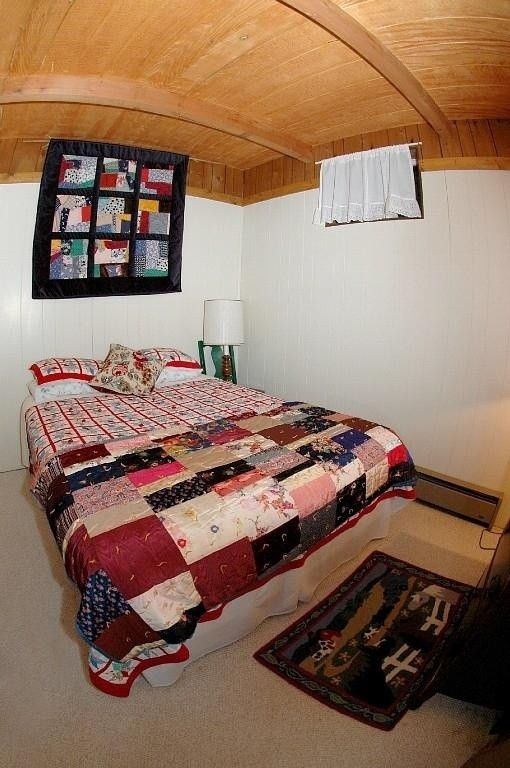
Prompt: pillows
29,343,204,403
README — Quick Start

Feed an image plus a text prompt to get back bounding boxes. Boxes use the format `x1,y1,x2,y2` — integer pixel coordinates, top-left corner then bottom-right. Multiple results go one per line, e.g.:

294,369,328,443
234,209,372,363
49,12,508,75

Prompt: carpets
253,550,482,736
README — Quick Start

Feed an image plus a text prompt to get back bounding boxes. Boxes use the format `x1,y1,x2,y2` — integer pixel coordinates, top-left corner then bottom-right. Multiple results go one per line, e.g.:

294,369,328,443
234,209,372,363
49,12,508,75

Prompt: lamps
201,297,248,384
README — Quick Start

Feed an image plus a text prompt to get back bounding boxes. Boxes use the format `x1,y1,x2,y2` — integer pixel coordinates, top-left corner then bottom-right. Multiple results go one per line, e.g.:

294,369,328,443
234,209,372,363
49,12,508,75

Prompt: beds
17,348,415,696
